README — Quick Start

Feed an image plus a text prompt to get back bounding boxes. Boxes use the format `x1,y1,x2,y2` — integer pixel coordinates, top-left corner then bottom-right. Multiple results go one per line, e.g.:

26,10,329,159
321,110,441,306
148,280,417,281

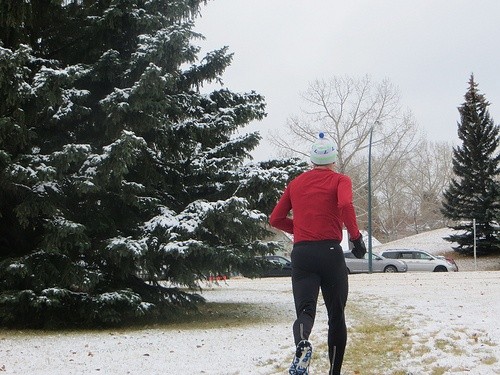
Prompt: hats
311,133,338,164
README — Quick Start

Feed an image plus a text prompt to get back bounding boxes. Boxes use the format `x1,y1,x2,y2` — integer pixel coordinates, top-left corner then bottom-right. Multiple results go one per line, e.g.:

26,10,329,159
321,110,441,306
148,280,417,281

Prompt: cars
254,255,293,278
380,250,459,272
342,249,408,275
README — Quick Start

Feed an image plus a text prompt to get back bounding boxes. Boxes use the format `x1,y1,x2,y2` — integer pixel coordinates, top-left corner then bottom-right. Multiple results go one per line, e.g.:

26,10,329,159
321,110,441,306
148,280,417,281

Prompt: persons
268,130,366,375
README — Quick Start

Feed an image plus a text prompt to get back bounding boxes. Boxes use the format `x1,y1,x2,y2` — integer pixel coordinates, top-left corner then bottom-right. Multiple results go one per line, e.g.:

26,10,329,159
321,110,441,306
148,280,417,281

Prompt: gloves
350,234,366,258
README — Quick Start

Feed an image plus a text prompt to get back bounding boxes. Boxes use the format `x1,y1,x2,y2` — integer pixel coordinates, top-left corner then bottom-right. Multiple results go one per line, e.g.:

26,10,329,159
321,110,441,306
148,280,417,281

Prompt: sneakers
289,340,312,375
